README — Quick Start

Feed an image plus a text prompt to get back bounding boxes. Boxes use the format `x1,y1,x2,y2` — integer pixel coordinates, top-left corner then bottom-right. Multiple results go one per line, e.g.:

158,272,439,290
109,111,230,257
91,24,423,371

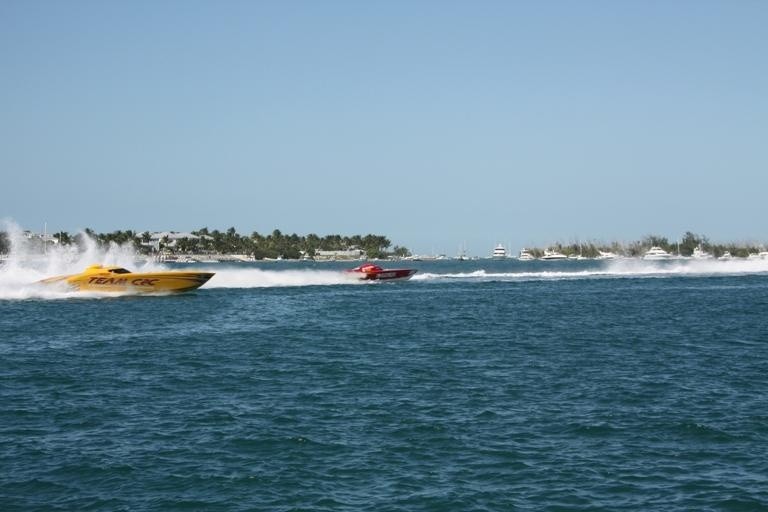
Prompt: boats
343,265,418,282
37,264,216,295
642,244,768,264
492,243,568,263
